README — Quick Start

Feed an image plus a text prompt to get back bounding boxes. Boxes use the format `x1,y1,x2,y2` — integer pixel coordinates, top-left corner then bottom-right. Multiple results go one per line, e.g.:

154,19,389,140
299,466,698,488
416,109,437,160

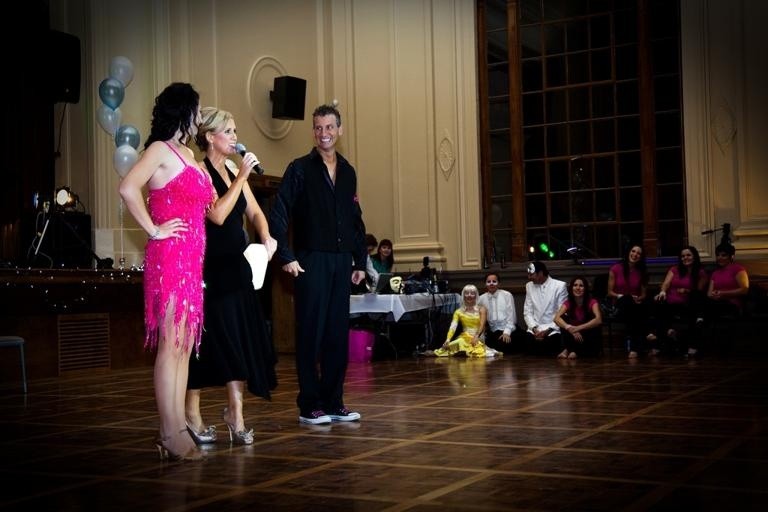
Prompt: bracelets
146,225,158,240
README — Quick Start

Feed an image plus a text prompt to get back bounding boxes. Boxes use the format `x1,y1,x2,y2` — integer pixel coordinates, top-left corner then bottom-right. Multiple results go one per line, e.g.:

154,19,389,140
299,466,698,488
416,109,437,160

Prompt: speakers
271,75,307,121
42,28,82,105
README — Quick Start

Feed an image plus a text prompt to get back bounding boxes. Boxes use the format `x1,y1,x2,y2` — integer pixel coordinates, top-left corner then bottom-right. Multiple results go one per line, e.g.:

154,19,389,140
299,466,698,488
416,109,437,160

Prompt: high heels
154,409,254,461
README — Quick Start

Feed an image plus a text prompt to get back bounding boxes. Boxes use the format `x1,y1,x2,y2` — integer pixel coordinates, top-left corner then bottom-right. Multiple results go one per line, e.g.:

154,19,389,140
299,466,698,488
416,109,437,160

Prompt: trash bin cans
347,324,375,363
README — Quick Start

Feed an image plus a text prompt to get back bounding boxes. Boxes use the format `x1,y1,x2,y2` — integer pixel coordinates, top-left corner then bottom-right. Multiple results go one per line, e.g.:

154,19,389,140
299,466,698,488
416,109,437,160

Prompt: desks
345,290,462,361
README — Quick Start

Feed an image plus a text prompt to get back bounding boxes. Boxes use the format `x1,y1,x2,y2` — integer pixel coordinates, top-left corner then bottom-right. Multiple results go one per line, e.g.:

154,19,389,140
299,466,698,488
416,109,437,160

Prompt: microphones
234,142,264,176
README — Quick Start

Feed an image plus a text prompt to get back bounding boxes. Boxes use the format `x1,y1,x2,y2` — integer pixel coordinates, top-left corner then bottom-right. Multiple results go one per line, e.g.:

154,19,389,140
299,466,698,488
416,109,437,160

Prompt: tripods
30,107,114,269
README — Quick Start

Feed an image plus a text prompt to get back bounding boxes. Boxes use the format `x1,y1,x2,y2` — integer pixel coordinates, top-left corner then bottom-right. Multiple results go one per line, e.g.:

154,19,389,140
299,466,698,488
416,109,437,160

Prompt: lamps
567,245,583,265
30,186,86,269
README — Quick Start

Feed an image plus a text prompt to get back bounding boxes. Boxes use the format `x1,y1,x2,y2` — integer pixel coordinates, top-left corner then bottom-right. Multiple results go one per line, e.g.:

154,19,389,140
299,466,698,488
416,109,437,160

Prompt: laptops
358,271,394,296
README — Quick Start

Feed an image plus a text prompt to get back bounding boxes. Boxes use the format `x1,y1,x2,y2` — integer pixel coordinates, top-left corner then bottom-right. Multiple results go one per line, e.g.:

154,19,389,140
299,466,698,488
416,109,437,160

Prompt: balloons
96,55,140,176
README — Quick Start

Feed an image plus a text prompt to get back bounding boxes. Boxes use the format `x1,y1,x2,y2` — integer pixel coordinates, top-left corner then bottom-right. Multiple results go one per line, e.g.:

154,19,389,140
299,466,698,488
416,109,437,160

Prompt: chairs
592,274,627,354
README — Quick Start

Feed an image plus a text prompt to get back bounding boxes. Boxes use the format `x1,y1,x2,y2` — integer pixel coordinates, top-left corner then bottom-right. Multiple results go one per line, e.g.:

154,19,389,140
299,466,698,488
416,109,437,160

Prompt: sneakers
299,407,361,424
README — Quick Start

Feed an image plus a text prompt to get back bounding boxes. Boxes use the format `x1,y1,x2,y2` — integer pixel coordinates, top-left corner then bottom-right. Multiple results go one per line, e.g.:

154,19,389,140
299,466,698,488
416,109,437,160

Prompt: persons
272,103,368,426
119,82,213,461
607,244,749,360
183,105,277,443
365,235,395,292
425,261,603,360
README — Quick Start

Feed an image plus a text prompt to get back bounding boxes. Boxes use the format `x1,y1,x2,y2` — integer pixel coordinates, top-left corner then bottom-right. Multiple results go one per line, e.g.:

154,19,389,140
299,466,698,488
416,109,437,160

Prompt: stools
0,335,31,394
